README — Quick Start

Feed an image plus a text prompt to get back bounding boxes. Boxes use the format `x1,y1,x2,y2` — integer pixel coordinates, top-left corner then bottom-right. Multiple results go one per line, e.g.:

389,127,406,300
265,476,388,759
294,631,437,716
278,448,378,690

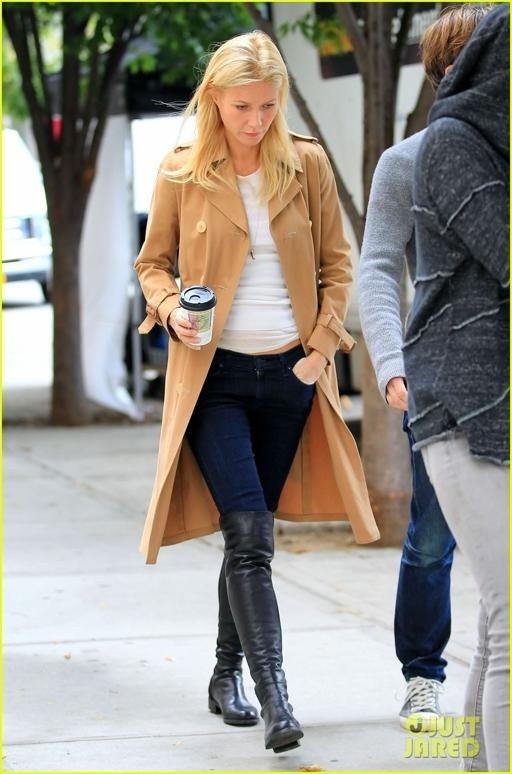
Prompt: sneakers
399,678,446,732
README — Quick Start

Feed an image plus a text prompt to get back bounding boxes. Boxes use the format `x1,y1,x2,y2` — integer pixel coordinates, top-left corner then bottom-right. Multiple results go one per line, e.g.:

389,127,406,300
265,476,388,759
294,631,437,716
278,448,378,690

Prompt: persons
133,30,355,753
355,2,497,734
407,2,507,773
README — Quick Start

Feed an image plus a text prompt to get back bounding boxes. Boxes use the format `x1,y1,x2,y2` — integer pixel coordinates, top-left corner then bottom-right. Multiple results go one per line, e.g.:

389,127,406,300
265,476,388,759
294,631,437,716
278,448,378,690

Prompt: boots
219,511,304,753
208,556,259,725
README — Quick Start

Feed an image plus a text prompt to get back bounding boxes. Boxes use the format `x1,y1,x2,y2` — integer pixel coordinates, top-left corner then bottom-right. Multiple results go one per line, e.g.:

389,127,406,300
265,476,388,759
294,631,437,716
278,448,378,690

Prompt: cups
177,284,216,347
